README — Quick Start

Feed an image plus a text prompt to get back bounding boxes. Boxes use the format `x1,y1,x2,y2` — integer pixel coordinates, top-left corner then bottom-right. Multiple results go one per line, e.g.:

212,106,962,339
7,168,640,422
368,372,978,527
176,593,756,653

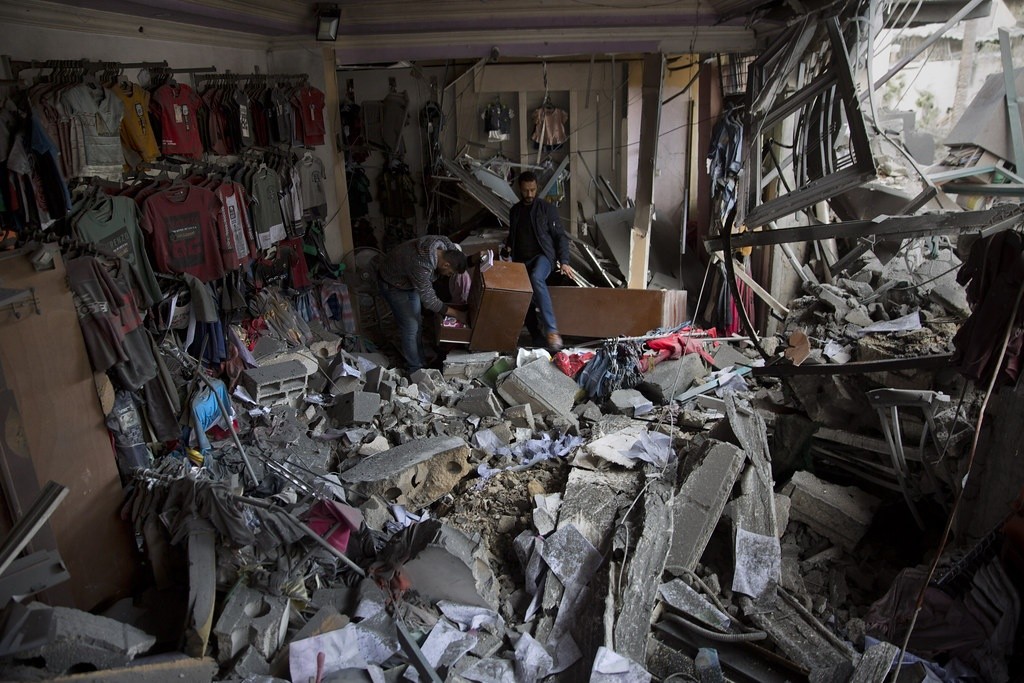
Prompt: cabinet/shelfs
432,260,533,355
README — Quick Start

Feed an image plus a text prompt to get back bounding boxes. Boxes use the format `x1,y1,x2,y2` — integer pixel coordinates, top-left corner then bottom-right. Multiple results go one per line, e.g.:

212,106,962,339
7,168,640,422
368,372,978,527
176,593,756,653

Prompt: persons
502,171,574,352
377,234,487,375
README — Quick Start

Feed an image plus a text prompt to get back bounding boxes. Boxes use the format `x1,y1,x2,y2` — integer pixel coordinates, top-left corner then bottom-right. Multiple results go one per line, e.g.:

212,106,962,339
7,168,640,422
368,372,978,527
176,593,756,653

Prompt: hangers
482,94,560,115
708,101,754,275
27,56,330,394
340,89,429,247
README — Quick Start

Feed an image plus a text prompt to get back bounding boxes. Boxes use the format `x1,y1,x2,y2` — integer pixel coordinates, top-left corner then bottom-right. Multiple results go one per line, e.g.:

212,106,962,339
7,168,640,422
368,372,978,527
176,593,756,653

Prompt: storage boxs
546,285,689,339
0,244,136,610
